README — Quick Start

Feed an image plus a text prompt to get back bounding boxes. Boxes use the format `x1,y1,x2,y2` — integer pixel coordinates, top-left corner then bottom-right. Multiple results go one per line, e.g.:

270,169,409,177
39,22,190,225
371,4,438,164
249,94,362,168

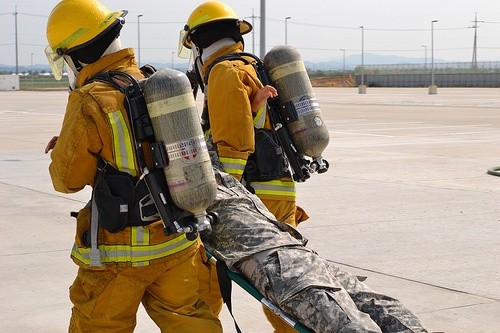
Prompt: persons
178,0,309,333
44,0,223,333
44,85,429,333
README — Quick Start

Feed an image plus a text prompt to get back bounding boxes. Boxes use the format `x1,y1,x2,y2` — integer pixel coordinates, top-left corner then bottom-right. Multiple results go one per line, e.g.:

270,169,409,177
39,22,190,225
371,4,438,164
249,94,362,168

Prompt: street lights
431,20,439,87
421,45,427,71
340,48,346,77
285,16,291,46
359,25,365,86
137,14,144,69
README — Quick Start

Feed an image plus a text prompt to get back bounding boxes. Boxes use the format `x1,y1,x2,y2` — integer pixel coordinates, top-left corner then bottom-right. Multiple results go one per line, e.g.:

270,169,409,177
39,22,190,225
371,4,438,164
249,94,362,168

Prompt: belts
245,246,313,279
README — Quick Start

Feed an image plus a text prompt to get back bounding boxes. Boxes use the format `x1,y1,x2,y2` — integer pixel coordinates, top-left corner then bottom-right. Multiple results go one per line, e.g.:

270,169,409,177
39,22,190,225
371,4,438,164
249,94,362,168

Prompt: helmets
182,0,252,48
46,0,127,62
